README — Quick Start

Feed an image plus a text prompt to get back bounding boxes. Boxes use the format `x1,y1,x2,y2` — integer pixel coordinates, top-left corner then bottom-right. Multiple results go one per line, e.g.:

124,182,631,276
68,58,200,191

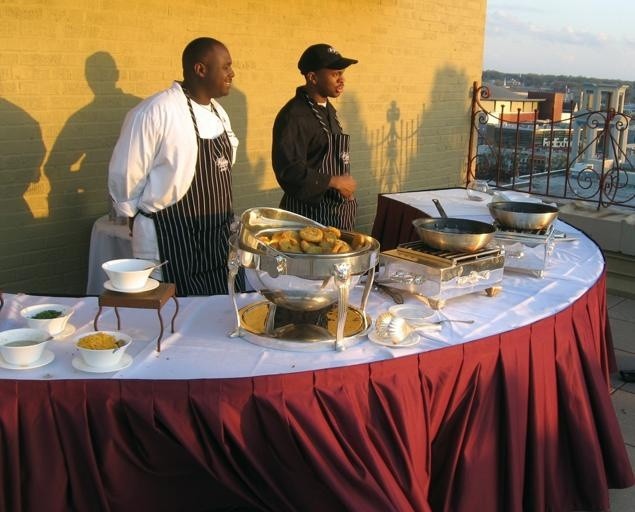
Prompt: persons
105,37,245,299
271,40,362,232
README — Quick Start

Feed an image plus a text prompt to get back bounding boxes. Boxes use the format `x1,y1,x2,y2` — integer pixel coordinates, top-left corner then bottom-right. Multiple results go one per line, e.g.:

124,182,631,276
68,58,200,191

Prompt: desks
86,210,132,295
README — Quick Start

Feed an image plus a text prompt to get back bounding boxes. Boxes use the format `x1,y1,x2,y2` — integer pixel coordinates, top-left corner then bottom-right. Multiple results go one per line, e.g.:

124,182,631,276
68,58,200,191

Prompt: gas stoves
489,222,557,280
378,240,505,310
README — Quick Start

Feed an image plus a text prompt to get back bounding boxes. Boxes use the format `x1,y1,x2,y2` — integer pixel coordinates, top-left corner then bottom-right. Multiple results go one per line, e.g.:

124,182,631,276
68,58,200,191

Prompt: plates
368,327,420,347
0,348,54,371
72,352,134,373
388,302,435,320
104,278,159,293
51,322,74,341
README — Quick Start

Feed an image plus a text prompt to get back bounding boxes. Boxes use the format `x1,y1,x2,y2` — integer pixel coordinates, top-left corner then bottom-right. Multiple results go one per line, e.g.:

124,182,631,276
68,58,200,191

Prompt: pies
259,226,353,253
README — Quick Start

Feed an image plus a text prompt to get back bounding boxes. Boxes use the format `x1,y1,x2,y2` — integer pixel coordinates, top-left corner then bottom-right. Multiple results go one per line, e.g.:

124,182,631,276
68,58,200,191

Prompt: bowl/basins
19,303,76,334
72,331,132,367
0,327,52,364
101,258,155,290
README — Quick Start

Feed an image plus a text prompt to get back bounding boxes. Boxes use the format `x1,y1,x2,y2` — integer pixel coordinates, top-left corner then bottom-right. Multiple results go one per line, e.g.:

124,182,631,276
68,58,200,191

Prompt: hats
298,44,359,74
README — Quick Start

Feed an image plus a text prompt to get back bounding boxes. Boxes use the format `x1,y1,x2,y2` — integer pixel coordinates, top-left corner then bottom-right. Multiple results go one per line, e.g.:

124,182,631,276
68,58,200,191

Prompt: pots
487,190,560,229
412,198,498,253
